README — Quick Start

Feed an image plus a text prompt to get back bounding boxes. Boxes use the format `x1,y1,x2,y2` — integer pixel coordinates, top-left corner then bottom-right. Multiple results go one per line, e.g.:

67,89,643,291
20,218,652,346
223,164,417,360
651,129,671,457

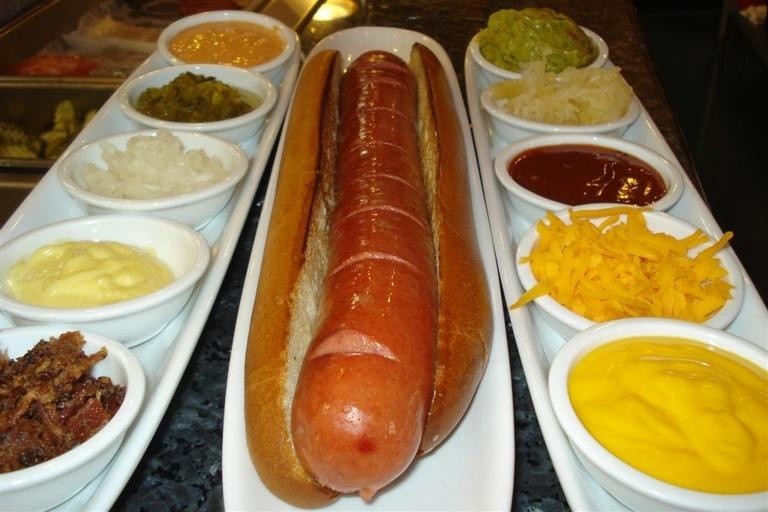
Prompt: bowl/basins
514,201,745,333
469,25,610,80
477,76,642,140
58,129,251,231
117,63,279,143
0,325,147,511
494,131,684,214
1,212,211,349
546,314,766,512
156,10,297,71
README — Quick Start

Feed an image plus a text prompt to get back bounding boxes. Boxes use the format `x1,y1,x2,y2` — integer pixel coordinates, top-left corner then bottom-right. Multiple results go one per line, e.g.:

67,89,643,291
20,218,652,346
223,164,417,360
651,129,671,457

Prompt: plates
2,9,302,510
465,25,766,511
218,23,521,511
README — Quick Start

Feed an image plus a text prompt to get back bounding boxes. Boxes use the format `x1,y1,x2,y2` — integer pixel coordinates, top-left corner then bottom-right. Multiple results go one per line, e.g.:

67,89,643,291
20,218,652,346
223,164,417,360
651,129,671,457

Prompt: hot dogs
242,42,494,511
